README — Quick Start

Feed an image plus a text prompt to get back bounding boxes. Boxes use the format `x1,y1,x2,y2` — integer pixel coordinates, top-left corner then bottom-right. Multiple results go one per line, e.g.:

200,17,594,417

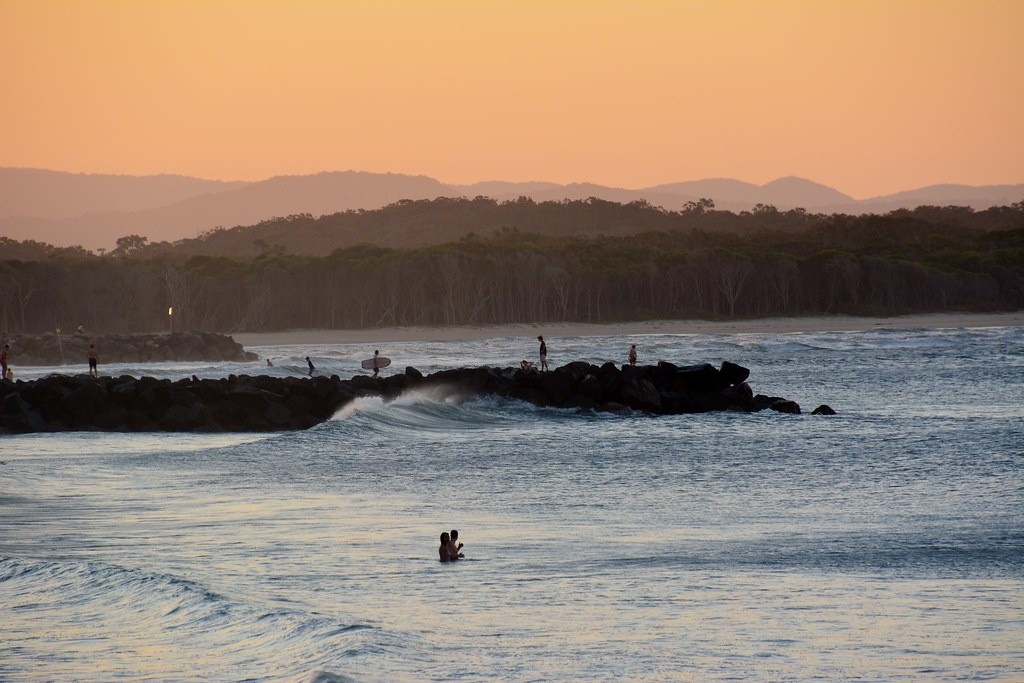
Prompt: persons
372,350,379,379
267,359,273,367
0,345,12,382
538,336,548,371
306,357,315,378
522,360,542,375
439,530,464,562
629,345,637,366
86,344,100,378
78,323,84,332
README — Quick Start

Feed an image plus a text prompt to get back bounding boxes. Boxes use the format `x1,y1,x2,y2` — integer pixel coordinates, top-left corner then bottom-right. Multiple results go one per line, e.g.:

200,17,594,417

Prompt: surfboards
361,357,392,369
